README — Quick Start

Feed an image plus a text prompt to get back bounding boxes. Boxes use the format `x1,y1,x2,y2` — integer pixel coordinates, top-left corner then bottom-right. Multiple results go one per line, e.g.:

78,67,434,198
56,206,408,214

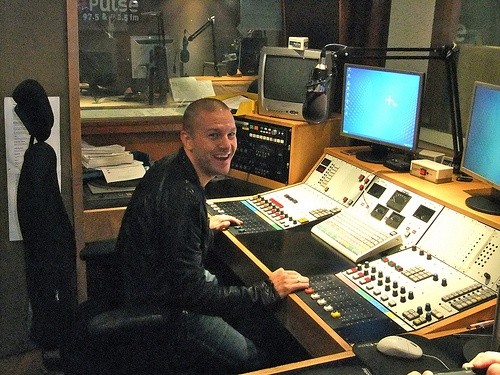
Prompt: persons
110,98,309,375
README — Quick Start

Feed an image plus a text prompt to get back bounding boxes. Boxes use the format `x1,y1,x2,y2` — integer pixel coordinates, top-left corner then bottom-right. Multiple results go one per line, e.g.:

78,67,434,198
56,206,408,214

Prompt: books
82,145,133,168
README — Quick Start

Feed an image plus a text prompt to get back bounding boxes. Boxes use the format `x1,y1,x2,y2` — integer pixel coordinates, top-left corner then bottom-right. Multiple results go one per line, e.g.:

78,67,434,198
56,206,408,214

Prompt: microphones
181,35,190,63
302,47,328,122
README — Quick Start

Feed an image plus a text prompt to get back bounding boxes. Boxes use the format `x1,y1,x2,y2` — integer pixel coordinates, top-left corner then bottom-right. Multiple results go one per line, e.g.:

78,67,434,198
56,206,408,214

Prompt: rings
297,278,299,282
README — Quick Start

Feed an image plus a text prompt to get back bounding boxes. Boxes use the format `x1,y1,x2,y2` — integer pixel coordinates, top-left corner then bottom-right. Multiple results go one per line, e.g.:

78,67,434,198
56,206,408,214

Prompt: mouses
377,335,423,359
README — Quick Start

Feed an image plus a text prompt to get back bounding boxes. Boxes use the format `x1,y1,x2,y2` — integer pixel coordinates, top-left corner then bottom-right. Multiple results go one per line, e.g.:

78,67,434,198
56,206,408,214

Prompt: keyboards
311,208,403,264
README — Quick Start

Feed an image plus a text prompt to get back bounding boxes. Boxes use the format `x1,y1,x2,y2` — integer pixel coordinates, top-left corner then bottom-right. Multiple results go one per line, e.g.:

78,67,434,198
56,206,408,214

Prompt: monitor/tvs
460,81,500,217
130,35,177,79
340,63,426,163
257,46,338,121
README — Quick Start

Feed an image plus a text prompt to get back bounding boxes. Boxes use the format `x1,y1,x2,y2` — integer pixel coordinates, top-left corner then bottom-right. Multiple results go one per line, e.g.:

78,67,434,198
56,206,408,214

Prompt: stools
60,291,183,375
